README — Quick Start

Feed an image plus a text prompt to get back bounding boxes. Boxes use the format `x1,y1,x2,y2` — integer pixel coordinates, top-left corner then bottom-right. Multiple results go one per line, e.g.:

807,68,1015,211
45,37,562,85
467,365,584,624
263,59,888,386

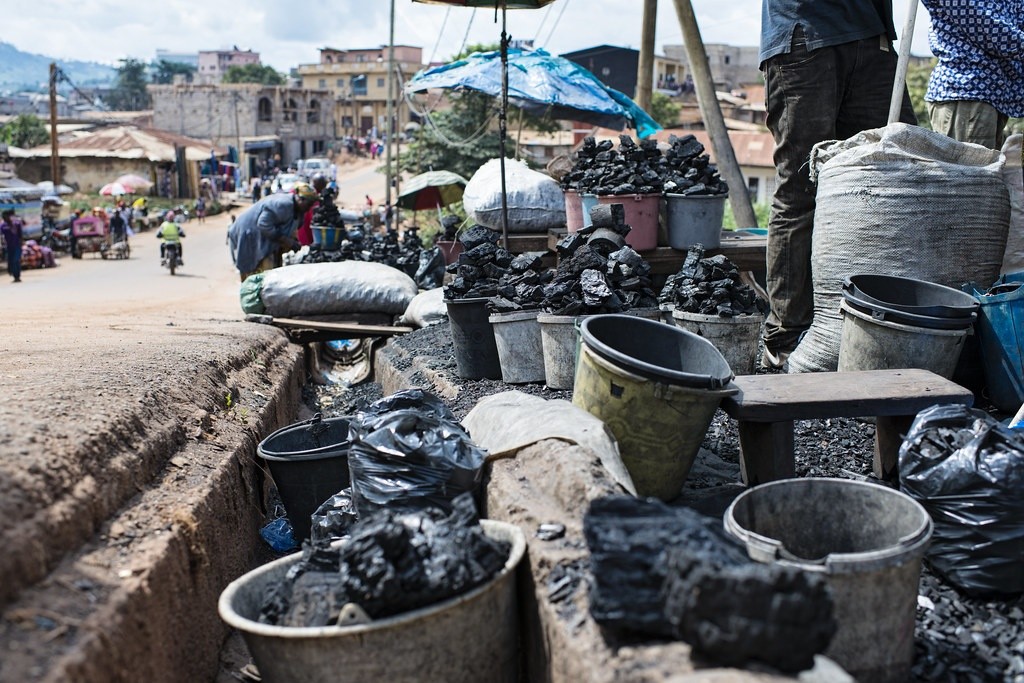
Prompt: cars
271,158,337,195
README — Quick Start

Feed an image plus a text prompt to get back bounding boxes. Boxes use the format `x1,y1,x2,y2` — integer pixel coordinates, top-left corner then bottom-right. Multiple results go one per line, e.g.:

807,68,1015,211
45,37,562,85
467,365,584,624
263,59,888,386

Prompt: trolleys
101,233,130,260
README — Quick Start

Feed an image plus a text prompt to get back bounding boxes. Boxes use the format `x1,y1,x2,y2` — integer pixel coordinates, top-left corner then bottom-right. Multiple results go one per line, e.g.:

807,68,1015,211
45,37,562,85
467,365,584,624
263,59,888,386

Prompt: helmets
167,211,175,221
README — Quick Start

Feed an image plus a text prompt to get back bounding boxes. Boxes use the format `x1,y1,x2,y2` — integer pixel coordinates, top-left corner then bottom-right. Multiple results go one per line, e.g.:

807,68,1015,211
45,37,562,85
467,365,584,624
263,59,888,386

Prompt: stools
717,369,973,490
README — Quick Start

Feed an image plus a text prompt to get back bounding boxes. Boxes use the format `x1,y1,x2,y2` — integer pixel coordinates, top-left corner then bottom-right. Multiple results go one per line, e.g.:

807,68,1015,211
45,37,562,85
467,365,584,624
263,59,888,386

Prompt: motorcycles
159,235,185,276
40,224,72,253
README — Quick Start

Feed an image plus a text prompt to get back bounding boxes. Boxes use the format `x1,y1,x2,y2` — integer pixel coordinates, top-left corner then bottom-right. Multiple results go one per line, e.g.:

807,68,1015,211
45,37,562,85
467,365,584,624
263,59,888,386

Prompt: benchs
548,226,767,283
494,232,548,254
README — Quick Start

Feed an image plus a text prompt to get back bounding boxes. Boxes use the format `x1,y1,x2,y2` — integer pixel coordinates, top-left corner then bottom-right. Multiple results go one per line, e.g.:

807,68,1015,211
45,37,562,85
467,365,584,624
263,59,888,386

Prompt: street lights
351,75,364,137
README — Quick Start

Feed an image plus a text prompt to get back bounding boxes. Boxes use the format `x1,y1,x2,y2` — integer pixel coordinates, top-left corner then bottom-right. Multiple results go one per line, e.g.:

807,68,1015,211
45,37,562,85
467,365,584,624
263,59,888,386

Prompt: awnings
0,177,72,199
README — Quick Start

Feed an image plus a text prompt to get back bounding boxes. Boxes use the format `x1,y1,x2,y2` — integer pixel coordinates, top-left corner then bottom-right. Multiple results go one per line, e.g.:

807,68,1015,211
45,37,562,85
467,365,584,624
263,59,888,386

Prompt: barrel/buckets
442,296,764,390
310,225,344,249
723,475,934,683
257,413,349,549
217,518,527,683
836,273,981,425
562,186,728,252
567,313,740,505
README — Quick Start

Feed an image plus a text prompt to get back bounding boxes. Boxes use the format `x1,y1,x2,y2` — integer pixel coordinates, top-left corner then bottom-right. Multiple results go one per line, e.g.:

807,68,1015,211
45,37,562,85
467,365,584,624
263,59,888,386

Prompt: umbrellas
99,182,136,195
118,174,154,201
402,47,662,159
395,165,469,228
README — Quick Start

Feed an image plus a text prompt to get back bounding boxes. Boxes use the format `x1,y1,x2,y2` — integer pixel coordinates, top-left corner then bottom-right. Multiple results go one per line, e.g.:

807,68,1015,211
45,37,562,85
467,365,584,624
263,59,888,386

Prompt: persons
0,128,387,282
921,0,1024,151
759,0,919,367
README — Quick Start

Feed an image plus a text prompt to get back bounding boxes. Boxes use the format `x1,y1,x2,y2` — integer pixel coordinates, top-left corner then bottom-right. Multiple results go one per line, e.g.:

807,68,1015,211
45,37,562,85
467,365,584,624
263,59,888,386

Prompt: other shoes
761,345,792,371
161,261,166,266
177,259,184,265
